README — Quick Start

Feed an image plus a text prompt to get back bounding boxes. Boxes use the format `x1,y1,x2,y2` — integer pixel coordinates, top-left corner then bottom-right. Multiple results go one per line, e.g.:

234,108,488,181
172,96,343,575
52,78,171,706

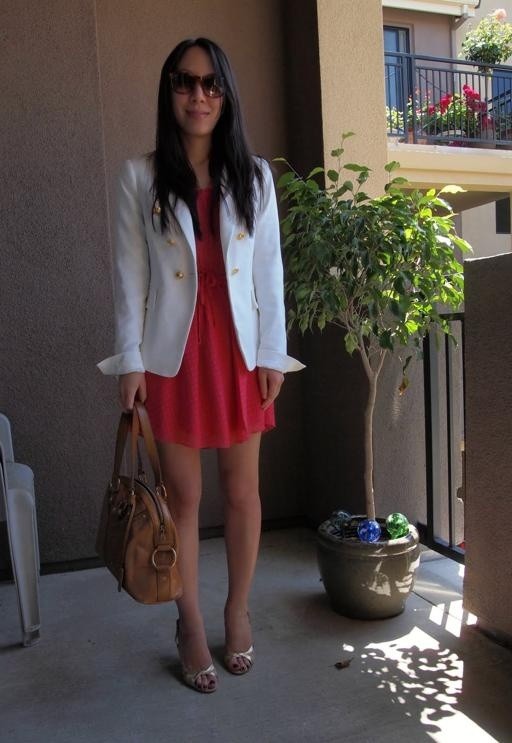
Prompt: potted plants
273,131,475,621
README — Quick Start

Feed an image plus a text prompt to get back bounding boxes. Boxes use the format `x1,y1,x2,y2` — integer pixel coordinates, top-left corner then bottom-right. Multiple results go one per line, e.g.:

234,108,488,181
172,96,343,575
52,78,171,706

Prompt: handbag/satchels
96,475,183,604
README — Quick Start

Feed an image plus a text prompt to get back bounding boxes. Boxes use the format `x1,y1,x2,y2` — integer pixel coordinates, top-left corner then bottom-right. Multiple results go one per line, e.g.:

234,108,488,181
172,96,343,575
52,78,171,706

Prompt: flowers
385,9,512,136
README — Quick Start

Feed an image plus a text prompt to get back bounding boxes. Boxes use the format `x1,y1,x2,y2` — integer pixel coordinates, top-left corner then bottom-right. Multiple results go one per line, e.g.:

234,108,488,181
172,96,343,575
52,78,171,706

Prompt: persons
95,36,309,695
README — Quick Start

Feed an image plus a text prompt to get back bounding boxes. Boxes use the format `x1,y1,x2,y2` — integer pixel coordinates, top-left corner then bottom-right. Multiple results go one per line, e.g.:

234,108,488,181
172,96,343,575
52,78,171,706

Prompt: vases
472,129,497,148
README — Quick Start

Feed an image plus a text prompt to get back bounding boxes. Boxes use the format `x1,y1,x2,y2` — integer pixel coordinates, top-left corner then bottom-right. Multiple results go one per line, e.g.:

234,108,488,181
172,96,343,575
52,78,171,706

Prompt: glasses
169,71,227,98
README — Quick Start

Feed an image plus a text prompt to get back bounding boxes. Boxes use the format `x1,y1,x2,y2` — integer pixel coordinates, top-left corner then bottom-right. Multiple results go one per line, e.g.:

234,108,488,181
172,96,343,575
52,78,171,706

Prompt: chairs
0,415,42,646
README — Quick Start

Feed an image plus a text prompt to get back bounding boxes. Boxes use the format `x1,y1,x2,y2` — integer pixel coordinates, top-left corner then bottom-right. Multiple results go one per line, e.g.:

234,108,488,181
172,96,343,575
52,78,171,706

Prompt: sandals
175,619,218,694
224,611,253,675
